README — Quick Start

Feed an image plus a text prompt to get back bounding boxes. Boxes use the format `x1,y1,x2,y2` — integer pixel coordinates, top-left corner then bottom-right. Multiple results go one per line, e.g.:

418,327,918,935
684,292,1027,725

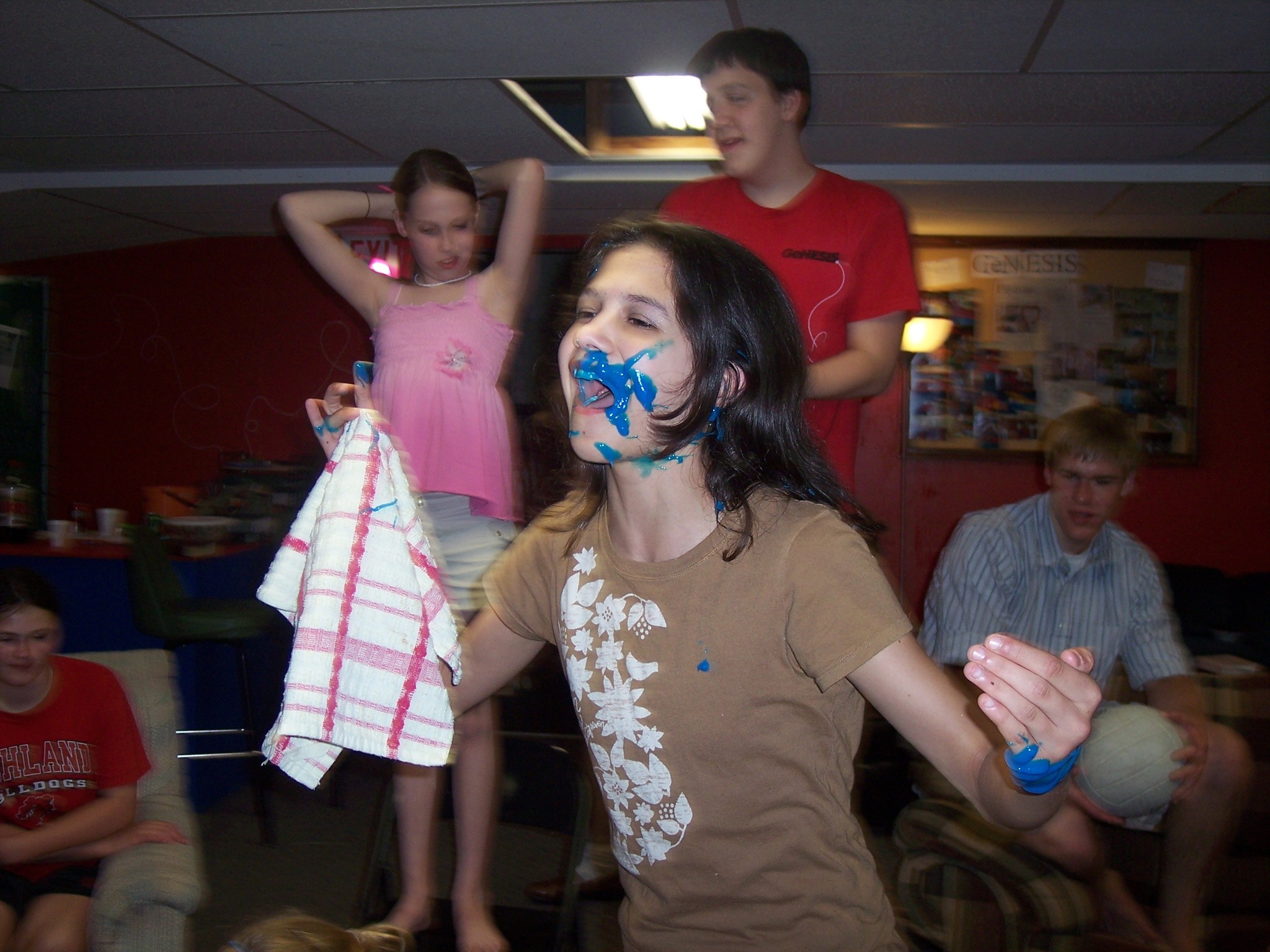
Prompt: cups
44,519,72,547
95,508,124,537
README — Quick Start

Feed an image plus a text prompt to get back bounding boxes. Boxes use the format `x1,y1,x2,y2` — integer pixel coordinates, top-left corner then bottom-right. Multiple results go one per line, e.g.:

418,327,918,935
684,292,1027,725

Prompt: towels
252,414,466,793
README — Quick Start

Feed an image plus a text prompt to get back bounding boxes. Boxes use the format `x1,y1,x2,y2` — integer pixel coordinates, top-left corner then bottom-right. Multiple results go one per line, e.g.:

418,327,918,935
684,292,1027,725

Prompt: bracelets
363,191,370,218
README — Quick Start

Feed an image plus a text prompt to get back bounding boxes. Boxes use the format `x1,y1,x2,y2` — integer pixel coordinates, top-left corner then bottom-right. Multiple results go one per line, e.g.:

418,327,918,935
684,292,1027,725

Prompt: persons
278,149,546,952
655,29,922,523
914,402,1255,951
0,567,191,952
305,214,1106,952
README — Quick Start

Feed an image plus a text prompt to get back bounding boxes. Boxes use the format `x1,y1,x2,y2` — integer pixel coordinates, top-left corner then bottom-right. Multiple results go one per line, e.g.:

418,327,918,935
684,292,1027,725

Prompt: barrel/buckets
140,486,201,519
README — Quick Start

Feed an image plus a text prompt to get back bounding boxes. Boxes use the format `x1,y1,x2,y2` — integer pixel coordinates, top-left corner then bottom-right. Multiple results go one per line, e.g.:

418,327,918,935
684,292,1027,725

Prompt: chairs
54,650,200,952
352,659,593,952
125,523,286,843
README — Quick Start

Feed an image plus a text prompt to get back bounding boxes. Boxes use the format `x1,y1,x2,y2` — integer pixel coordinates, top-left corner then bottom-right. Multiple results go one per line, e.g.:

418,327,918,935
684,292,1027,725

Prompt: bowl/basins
160,516,241,557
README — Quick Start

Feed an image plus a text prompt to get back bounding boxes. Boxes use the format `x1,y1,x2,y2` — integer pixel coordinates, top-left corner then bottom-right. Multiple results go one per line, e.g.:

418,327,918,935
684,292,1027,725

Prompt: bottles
0,459,33,544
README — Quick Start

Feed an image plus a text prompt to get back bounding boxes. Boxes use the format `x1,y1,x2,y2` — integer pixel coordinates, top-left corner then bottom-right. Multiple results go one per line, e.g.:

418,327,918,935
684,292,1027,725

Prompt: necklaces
414,272,473,289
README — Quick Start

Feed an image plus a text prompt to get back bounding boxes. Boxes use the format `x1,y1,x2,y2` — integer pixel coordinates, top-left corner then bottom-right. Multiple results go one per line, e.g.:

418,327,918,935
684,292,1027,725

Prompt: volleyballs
1074,698,1194,820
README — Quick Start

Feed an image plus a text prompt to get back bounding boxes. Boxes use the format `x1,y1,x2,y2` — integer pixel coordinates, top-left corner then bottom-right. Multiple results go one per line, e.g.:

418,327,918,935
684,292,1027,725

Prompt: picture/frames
904,235,1203,465
